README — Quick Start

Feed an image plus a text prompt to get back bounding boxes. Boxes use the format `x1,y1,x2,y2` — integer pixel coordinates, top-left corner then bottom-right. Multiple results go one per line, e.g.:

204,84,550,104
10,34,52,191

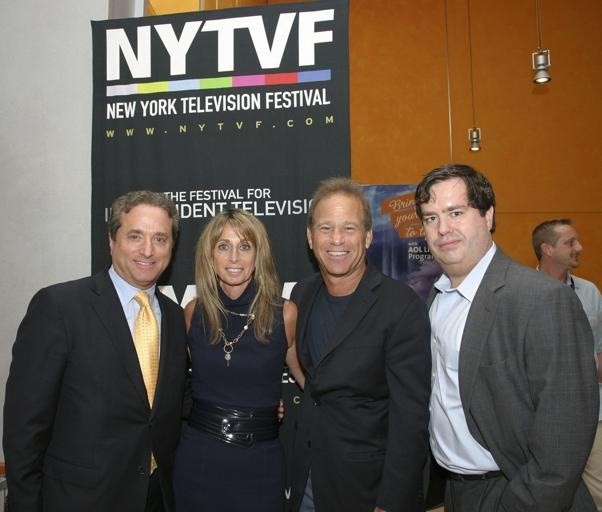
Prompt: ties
131,291,160,477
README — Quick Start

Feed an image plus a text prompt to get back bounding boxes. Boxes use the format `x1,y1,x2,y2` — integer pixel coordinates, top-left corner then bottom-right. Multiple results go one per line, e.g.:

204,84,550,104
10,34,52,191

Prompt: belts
449,469,505,482
187,404,279,448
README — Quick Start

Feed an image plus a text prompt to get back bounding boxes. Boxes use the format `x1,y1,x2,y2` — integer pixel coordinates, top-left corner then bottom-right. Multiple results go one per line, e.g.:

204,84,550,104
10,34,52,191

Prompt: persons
170,208,306,512
532,217,602,512
415,164,599,512
286,176,431,512
2,190,284,512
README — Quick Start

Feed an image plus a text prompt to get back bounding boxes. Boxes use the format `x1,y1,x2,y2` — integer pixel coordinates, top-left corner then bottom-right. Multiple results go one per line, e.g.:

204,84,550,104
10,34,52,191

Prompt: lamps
530,1,552,84
464,5,481,155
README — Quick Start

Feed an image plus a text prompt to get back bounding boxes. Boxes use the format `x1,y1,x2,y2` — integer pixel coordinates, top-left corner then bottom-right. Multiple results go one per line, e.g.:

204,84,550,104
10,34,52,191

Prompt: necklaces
217,312,257,368
